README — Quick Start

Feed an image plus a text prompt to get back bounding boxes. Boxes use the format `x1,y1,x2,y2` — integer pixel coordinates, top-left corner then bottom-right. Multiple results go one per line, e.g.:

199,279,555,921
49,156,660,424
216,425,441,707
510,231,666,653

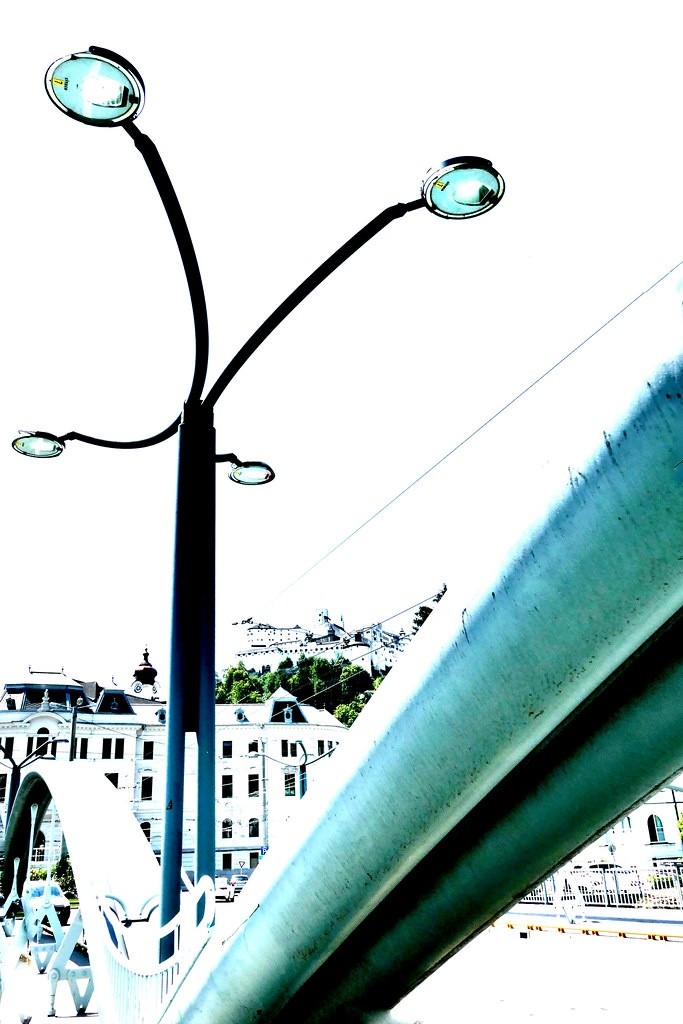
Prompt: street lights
5,38,506,1005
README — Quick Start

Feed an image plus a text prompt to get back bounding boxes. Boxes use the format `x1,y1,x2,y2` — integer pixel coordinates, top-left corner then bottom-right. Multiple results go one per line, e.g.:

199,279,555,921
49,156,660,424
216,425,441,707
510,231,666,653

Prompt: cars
564,862,644,902
29,879,71,927
214,877,234,901
230,874,249,893
